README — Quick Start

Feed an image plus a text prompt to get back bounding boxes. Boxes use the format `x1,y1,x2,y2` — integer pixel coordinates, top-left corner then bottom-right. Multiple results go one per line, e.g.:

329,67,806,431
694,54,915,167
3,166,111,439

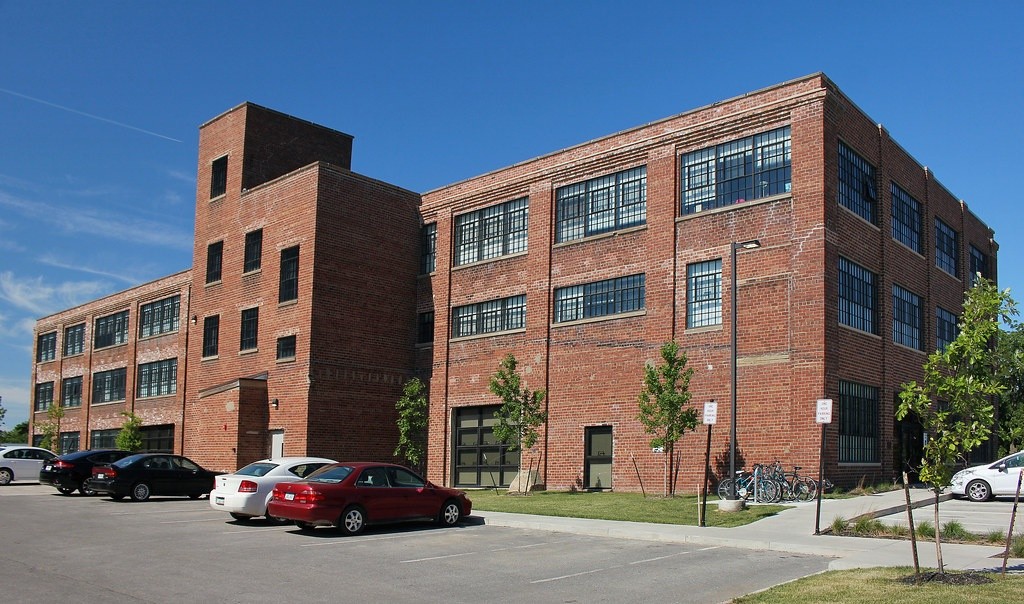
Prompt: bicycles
717,461,816,503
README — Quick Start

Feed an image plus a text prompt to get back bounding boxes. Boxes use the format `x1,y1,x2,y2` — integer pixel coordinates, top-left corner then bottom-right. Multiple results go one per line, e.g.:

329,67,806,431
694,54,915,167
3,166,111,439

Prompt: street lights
717,239,761,510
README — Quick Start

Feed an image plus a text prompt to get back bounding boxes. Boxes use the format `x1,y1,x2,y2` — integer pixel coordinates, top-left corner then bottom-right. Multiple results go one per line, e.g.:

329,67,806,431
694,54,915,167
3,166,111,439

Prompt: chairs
151,461,170,468
356,472,393,487
255,469,264,475
17,451,32,459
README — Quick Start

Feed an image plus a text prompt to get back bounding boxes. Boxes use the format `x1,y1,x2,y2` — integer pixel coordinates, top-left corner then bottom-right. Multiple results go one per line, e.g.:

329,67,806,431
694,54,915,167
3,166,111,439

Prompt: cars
268,462,472,536
0,446,58,486
950,450,1024,502
210,457,373,525
39,446,150,497
87,453,228,502
